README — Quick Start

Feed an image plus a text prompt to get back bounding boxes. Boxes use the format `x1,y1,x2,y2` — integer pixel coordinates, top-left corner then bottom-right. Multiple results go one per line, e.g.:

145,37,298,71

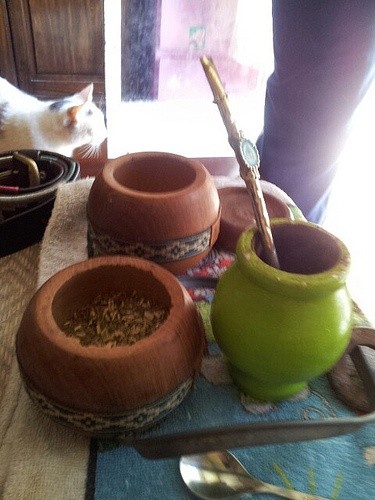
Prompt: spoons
179,449,328,500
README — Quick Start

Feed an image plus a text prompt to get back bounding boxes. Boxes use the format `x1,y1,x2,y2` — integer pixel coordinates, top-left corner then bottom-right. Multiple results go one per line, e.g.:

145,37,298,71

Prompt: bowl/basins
85,152,222,274
16,255,205,435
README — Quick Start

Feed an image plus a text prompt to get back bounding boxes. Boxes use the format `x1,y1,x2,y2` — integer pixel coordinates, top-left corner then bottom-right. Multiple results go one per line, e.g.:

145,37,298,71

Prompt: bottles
210,218,353,403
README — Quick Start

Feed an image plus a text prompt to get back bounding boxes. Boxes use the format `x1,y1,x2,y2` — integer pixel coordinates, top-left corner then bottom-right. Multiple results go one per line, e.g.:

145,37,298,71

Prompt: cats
0,76,110,160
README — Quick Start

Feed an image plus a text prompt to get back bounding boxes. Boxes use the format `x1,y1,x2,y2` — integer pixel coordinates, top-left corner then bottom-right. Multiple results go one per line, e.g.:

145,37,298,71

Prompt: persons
258,0,375,225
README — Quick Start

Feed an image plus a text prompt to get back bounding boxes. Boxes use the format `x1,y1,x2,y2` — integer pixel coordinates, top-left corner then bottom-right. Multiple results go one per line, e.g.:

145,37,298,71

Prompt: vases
211,219,352,402
14,255,205,442
84,151,224,277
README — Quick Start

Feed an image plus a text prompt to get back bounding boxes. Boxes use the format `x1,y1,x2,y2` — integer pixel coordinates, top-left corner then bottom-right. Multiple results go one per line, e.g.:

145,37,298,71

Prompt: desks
1,176,375,500
